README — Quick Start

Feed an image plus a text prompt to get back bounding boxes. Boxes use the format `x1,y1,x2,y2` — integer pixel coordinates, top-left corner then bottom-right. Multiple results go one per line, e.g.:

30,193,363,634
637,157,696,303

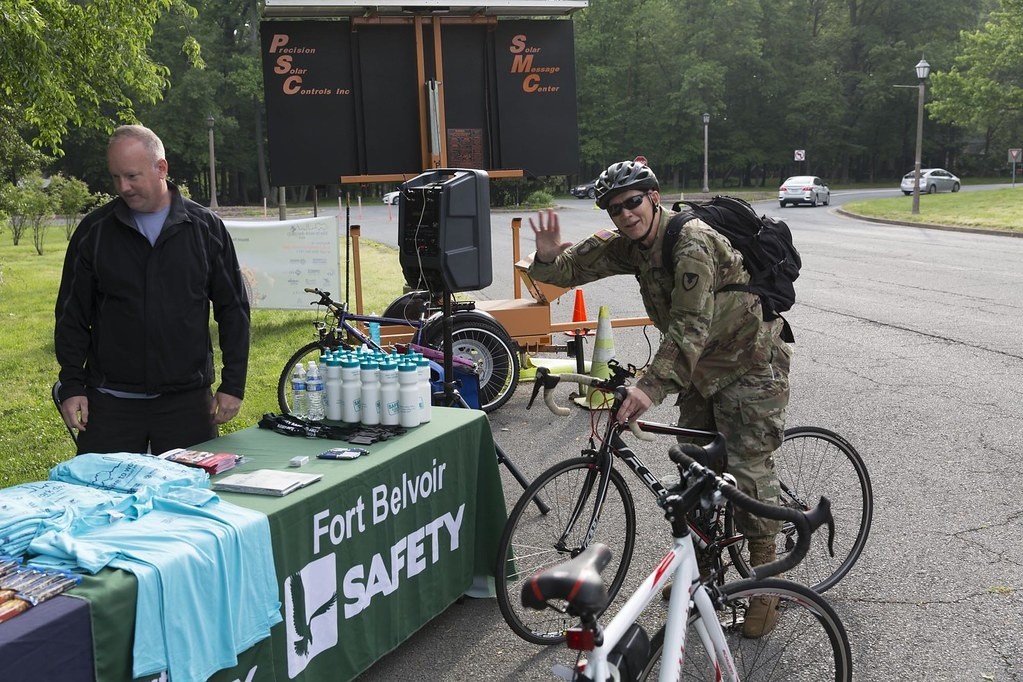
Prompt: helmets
593,161,660,210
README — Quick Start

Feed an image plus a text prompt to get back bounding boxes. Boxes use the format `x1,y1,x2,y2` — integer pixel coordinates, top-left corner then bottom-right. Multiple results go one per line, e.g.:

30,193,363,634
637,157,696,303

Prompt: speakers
398,168,493,293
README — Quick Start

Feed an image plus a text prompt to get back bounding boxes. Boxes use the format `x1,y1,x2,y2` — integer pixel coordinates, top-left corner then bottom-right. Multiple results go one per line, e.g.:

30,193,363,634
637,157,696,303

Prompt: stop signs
634,155,647,166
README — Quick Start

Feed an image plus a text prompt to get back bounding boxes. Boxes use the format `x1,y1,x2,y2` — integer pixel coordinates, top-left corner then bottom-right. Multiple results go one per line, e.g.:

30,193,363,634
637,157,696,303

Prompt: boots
741,543,780,639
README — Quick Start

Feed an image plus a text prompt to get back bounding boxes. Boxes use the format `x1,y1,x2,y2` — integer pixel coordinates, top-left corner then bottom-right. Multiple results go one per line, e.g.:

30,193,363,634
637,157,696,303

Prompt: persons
54,125,251,456
529,161,793,639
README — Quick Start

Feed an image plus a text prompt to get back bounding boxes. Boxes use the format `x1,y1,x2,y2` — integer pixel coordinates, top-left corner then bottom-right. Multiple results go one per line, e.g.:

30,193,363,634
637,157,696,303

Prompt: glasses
607,193,648,217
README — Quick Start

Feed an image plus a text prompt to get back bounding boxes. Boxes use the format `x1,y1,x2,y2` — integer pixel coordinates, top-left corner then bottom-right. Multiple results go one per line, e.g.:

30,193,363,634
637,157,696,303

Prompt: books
211,469,324,496
157,448,244,475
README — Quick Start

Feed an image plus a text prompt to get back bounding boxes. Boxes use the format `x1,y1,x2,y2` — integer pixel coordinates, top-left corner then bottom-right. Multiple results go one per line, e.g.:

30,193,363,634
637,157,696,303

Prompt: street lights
702,112,711,192
912,58,931,215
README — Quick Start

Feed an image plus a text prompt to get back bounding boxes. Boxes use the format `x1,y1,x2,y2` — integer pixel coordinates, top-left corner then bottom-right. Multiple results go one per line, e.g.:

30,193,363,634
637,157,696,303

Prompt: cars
900,168,962,195
777,175,830,207
569,177,595,199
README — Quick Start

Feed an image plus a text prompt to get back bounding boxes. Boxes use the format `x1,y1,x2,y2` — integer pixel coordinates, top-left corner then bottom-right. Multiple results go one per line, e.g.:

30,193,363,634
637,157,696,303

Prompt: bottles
319,345,431,428
291,361,326,419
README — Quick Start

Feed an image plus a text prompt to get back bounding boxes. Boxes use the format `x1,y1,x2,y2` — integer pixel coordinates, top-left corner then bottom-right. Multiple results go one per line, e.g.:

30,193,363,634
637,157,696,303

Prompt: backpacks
662,196,802,343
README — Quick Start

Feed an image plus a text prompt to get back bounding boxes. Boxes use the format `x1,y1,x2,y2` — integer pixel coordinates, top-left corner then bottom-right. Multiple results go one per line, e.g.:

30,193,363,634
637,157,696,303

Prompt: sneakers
662,569,712,600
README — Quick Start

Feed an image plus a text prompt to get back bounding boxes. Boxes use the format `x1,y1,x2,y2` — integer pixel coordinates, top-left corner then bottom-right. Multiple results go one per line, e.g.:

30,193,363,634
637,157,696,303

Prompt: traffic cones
564,289,595,337
573,306,617,410
506,348,592,386
592,197,599,209
679,192,686,206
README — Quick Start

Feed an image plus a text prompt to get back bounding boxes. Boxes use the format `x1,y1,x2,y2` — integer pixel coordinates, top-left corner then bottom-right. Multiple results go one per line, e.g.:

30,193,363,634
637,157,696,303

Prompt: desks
0,406,520,682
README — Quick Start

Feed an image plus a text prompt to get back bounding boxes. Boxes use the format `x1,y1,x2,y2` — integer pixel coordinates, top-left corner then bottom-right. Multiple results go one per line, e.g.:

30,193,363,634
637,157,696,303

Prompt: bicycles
277,287,521,414
494,358,873,646
521,442,853,682
